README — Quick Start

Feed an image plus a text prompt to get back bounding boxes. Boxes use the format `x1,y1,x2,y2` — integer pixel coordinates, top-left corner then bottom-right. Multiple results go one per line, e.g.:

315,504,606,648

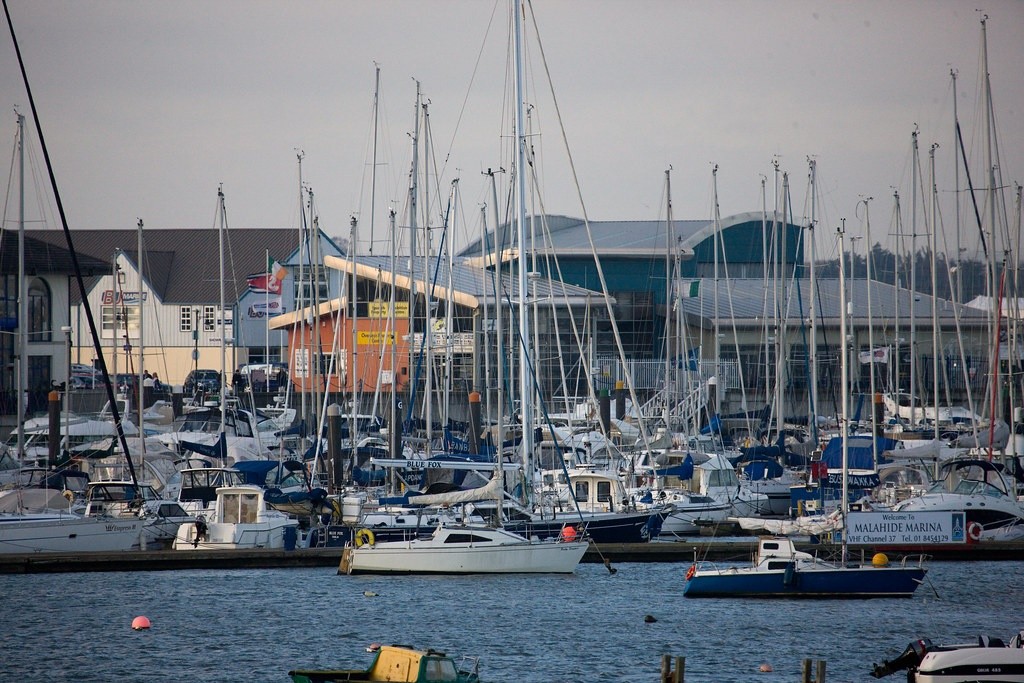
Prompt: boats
288,644,487,683
867,632,1024,683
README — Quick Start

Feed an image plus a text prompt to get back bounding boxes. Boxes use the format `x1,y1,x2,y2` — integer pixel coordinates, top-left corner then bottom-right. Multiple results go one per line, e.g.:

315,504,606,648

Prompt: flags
246,272,281,295
671,346,700,373
267,255,289,280
683,280,700,299
858,347,889,364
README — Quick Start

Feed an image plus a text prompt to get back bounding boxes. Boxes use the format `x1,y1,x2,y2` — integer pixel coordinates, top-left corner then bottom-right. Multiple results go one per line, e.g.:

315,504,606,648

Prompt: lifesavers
686,566,695,580
968,522,984,540
798,500,803,516
401,483,404,492
62,489,74,503
745,440,750,448
332,501,339,517
354,528,375,547
789,506,792,517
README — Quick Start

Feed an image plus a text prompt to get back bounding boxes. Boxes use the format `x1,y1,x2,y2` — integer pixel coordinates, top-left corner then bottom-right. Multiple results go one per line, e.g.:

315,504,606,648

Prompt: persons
220,370,227,382
277,369,287,387
143,370,162,390
232,370,242,386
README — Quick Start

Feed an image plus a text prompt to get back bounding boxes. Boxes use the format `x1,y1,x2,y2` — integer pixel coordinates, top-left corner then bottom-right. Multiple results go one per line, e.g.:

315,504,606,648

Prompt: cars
117,371,173,394
182,369,221,395
69,375,120,390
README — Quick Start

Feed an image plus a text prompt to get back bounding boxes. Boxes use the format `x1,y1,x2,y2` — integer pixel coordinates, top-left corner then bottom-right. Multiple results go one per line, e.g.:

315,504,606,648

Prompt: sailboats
682,216,934,599
2,0,1024,576
